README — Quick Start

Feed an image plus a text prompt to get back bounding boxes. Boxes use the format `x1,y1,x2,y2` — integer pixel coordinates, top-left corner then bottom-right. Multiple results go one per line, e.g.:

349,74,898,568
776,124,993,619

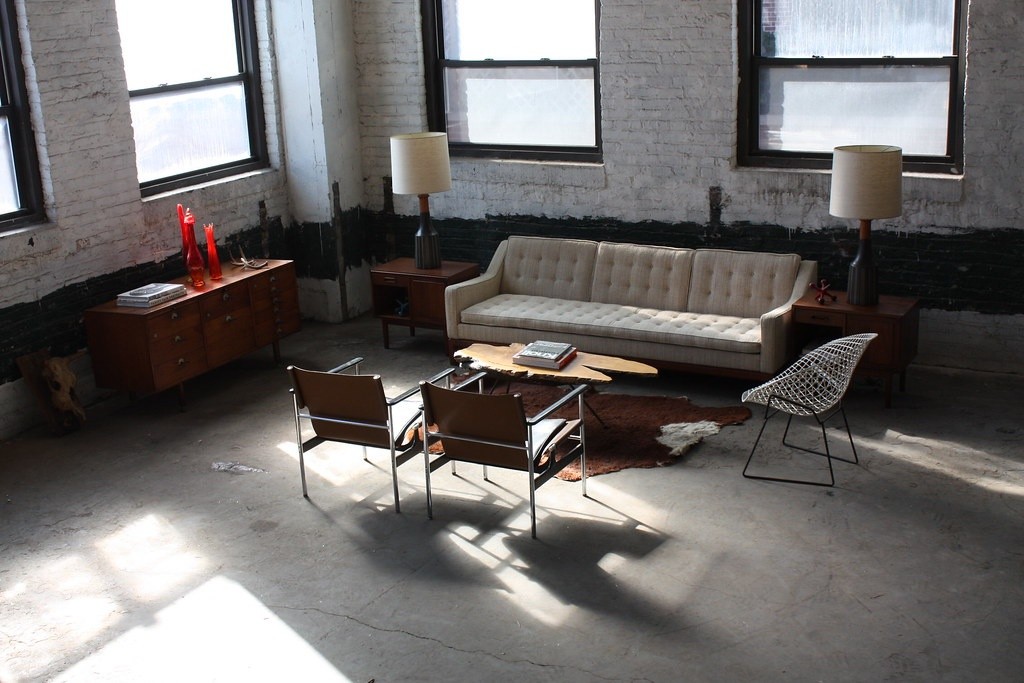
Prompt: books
116,283,187,307
513,340,577,369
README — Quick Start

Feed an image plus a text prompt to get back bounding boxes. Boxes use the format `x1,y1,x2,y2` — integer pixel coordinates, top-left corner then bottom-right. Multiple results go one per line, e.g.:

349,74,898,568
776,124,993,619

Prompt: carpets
406,381,753,480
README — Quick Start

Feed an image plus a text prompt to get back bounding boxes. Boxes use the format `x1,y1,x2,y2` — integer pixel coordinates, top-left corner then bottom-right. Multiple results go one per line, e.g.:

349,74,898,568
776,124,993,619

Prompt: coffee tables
453,342,658,428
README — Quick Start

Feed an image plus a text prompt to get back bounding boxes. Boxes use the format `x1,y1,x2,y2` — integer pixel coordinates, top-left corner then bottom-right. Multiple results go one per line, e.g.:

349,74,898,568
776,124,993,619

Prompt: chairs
286,356,456,513
741,332,878,487
419,372,591,539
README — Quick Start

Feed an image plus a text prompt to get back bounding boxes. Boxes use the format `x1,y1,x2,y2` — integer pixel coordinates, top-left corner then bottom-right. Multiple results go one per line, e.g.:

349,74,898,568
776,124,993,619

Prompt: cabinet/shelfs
83,257,302,413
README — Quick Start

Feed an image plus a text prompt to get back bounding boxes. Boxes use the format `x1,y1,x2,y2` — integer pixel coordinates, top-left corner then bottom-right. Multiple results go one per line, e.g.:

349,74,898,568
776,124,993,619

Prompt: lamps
829,145,903,306
389,132,453,270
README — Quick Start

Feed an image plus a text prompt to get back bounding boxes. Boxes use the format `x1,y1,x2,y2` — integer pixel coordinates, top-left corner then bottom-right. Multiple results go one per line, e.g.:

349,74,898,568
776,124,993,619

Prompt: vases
177,203,195,283
203,222,223,280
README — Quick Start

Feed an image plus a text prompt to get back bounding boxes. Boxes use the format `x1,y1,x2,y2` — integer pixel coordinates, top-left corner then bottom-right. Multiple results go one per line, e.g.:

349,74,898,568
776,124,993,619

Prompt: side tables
788,289,921,408
370,256,480,356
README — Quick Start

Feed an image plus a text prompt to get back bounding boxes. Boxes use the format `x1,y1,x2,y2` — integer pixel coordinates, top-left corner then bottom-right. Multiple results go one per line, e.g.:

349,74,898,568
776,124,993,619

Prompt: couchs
444,235,818,391
